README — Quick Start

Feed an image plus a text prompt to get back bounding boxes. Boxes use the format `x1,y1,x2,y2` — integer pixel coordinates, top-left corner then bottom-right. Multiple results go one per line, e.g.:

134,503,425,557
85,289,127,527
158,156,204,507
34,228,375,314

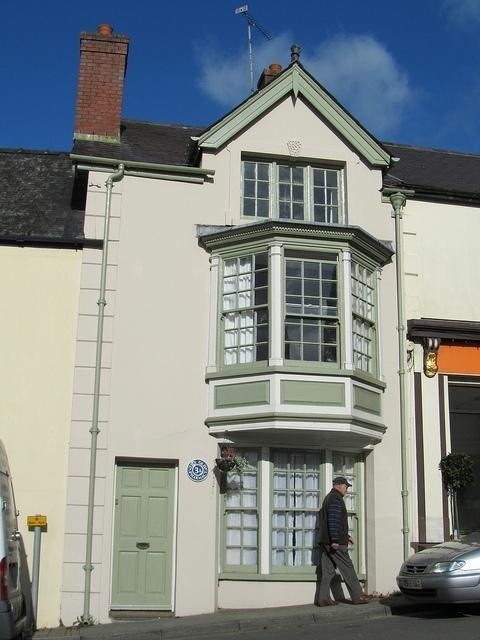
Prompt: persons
315,476,373,606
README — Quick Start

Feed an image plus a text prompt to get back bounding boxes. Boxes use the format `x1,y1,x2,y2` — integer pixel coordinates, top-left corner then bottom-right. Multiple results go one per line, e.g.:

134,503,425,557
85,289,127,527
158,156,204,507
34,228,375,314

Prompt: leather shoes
319,598,338,607
352,594,370,604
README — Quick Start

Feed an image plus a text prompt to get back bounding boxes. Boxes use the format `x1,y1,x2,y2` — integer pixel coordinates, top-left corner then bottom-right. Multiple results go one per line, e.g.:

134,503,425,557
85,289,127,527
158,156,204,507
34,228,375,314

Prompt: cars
396,530,480,605
0,439,36,640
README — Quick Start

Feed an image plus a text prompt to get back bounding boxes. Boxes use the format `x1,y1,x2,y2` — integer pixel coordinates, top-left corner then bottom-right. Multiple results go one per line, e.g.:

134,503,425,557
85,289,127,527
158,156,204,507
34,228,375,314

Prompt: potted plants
215,430,248,472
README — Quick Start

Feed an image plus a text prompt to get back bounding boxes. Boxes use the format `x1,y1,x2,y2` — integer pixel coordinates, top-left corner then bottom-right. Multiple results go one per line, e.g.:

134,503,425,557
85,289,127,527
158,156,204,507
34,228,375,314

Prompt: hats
332,476,352,487
